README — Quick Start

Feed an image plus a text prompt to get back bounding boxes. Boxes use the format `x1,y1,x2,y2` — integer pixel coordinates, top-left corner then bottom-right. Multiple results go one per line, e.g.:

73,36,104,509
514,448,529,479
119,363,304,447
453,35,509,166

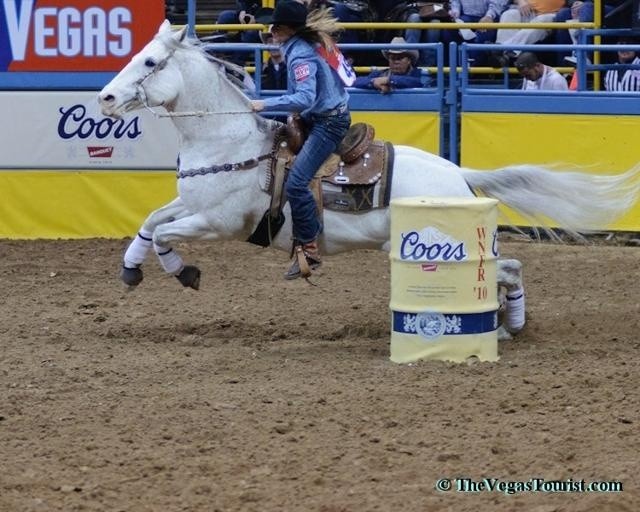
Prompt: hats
255,0,307,26
381,37,419,63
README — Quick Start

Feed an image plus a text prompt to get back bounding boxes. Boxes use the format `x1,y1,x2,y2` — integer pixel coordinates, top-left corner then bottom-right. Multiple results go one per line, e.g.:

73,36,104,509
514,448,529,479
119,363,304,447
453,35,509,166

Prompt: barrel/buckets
389,198,500,367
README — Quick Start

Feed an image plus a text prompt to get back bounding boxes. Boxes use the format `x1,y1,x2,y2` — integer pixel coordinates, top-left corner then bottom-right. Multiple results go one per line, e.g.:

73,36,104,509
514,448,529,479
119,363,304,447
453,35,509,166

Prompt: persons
212,2,639,92
245,0,353,282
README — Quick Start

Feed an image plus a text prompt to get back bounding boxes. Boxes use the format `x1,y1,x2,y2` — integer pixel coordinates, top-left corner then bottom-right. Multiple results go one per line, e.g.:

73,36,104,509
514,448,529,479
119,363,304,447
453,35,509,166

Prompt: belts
312,104,347,117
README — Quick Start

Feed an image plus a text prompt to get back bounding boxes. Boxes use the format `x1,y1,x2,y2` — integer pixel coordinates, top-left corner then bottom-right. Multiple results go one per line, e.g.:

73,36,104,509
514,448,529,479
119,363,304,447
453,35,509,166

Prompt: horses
95,15,640,346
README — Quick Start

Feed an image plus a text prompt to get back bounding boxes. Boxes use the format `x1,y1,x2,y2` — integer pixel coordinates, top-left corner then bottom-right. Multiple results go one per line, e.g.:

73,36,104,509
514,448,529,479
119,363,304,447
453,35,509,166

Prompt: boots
283,241,322,280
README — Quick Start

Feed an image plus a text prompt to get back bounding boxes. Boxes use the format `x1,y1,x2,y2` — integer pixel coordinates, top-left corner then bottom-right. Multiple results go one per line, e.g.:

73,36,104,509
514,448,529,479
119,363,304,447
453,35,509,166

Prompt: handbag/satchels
420,6,450,18
